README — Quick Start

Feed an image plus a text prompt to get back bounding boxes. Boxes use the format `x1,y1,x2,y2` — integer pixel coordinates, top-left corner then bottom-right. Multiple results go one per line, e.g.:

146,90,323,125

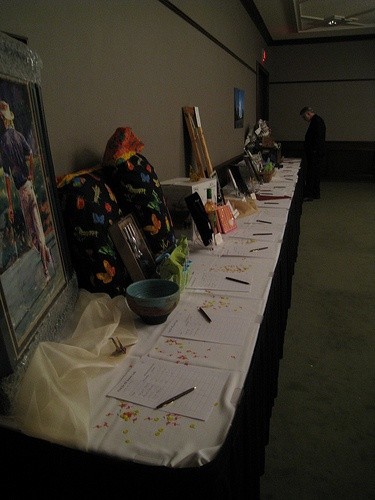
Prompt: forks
124,222,151,278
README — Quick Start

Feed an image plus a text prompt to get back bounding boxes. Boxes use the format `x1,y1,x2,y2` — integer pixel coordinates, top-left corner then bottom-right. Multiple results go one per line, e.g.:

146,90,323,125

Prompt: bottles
204,188,216,231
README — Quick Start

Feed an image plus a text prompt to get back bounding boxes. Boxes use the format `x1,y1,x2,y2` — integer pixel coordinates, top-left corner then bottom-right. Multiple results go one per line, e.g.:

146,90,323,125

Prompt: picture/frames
0,30,79,377
182,107,226,206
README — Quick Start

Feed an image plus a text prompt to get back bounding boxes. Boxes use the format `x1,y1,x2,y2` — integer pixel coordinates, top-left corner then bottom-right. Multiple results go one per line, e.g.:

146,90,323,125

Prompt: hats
0,101,15,121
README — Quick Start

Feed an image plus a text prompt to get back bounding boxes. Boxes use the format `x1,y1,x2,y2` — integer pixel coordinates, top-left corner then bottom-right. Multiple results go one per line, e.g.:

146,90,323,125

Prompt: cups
189,162,201,182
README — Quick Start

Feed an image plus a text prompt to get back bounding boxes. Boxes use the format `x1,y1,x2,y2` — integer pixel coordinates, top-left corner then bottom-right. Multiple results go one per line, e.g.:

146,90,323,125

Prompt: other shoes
47,248,54,269
46,272,51,282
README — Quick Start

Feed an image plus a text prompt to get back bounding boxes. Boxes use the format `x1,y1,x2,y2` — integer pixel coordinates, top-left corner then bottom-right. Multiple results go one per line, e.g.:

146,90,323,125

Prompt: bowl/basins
126,279,180,325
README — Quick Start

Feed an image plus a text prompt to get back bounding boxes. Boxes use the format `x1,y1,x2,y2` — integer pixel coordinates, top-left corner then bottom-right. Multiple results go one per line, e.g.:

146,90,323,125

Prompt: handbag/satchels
55,126,178,298
157,235,192,289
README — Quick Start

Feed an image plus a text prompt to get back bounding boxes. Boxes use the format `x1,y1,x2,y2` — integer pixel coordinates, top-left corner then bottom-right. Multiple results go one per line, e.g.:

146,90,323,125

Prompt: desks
0,157,303,500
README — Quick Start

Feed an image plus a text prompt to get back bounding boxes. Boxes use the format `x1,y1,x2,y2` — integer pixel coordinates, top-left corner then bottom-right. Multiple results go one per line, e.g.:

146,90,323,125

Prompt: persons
0,100,55,282
299,106,326,201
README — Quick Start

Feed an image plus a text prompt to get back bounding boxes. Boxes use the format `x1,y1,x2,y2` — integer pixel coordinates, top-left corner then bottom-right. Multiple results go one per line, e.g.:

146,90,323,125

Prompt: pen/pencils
249,246,269,252
263,202,281,204
252,233,272,235
224,276,251,286
255,220,272,224
273,186,287,189
260,192,274,195
149,387,200,411
260,189,273,191
197,307,213,324
